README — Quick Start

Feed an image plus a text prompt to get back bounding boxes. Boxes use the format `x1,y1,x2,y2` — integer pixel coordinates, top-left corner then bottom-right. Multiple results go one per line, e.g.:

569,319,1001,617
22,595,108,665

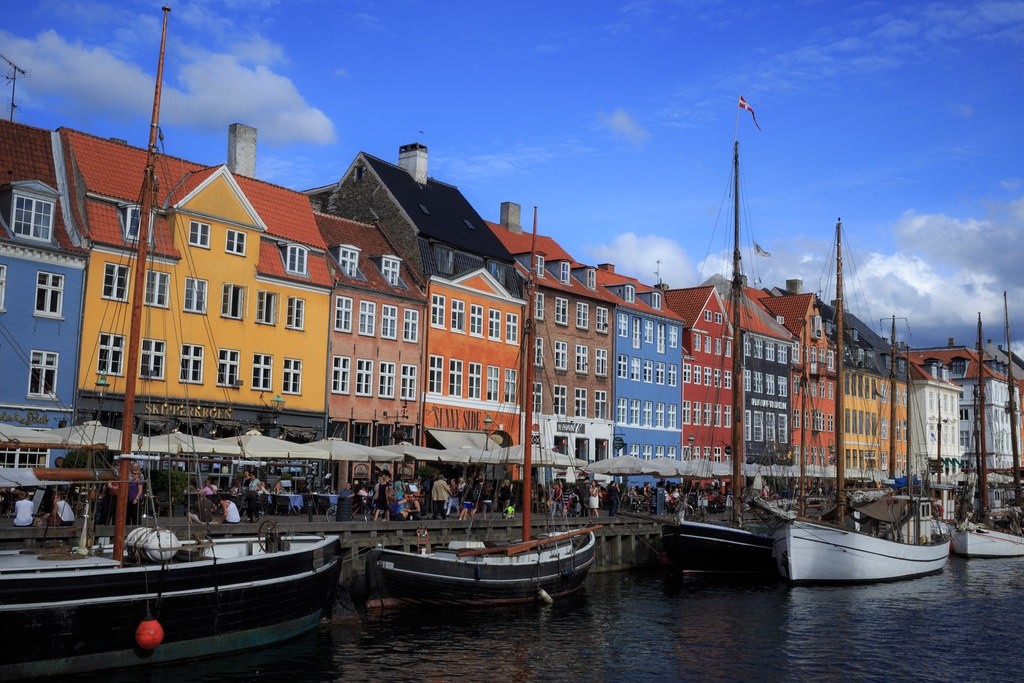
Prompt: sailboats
949,311,1024,556
365,205,604,615
775,215,950,581
661,139,774,577
0,6,343,683
748,290,1024,526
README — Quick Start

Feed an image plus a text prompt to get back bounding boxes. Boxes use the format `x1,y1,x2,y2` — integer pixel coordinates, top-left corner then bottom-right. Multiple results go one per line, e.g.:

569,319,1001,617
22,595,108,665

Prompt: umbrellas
846,468,1024,484
585,455,838,478
0,421,588,468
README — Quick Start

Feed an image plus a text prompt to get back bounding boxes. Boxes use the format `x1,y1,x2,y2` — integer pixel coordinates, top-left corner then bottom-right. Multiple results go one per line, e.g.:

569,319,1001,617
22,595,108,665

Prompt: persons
90,462,145,525
532,477,733,522
813,482,822,495
13,492,35,527
183,470,309,524
340,467,517,520
44,494,75,526
761,482,800,499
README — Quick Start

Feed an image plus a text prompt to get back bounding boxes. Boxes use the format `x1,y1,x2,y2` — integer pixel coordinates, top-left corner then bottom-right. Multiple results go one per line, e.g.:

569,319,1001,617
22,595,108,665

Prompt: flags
755,243,771,257
740,96,761,131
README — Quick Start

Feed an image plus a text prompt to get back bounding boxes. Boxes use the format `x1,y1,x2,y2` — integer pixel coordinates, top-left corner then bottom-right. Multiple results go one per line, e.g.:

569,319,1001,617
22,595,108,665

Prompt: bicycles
326,498,377,523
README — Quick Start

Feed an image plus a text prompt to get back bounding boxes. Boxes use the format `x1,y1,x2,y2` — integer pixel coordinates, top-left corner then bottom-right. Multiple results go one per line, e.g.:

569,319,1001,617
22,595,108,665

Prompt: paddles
455,524,604,558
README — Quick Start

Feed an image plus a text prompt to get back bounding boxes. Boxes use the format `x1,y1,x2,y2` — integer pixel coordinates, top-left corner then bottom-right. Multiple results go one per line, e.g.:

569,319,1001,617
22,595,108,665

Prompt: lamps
256,391,286,422
94,368,110,404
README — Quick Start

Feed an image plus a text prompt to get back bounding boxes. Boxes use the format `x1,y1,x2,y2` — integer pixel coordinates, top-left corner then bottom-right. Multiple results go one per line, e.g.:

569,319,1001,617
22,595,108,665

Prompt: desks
260,493,303,517
311,493,339,515
185,493,228,514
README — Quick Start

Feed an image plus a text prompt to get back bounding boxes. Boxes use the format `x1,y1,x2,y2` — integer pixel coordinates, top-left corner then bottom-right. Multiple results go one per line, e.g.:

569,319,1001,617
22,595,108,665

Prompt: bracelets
135,498,138,500
